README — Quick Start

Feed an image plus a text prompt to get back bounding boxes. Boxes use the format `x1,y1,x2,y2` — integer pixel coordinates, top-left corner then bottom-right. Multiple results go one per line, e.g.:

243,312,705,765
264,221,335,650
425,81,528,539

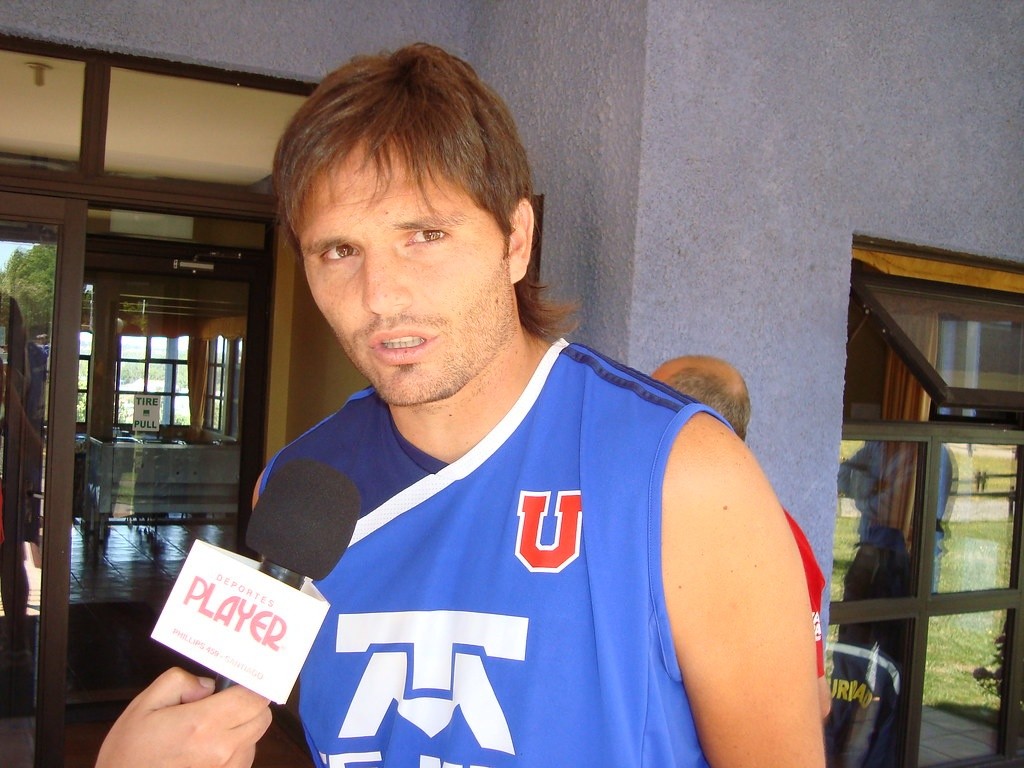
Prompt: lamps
109,209,194,240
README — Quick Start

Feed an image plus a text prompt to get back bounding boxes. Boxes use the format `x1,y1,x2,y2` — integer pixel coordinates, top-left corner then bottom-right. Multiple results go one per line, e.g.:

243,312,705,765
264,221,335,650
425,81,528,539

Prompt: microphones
151,457,361,706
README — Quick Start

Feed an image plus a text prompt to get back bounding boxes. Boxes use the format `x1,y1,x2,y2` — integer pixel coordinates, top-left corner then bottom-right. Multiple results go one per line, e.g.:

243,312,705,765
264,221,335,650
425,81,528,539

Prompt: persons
833,439,952,768
650,355,832,725
0,294,48,667
252,42,827,768
95,667,272,768
